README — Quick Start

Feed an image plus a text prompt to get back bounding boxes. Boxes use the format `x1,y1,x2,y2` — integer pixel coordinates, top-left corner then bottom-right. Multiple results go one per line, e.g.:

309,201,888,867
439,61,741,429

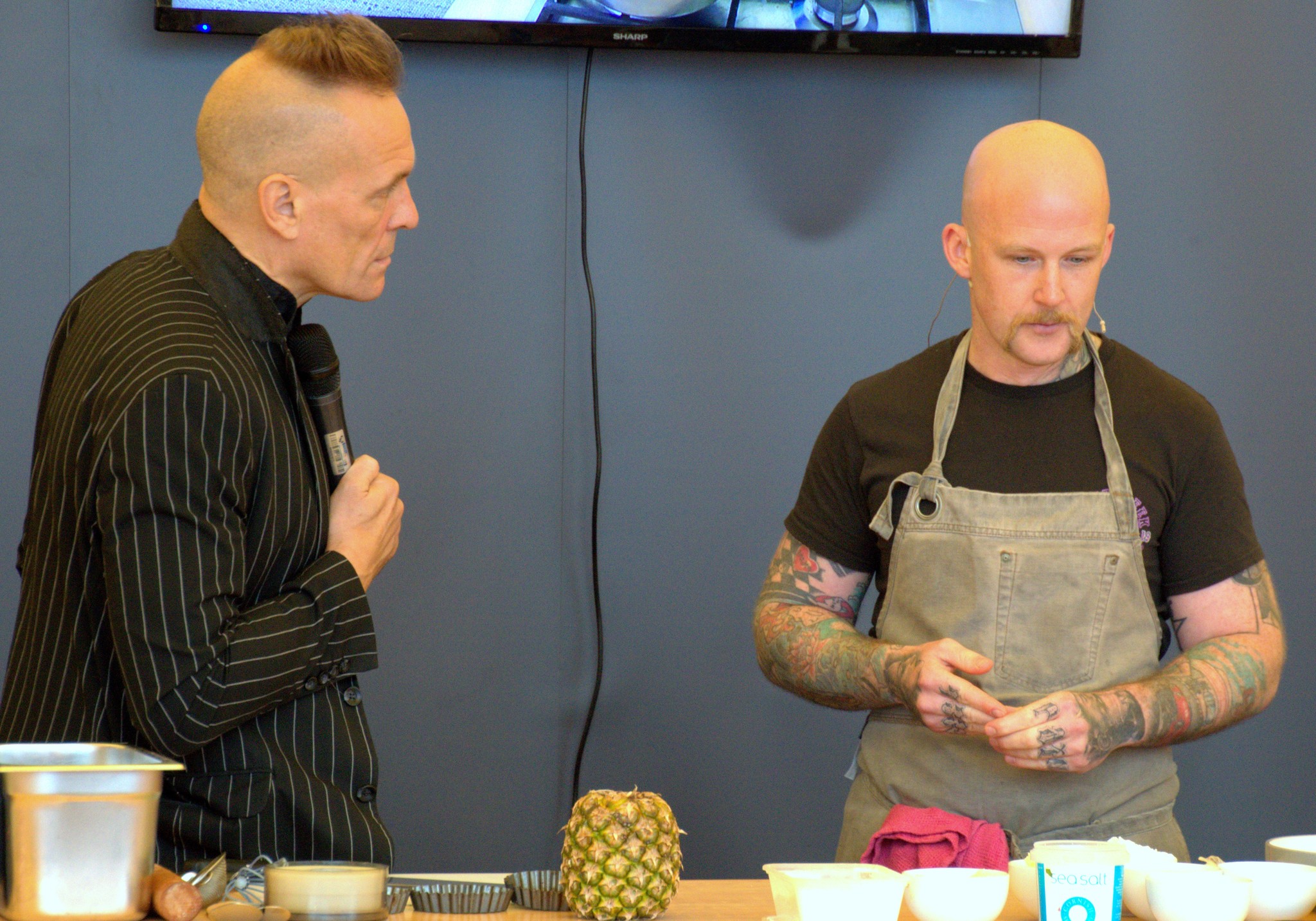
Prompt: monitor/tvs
151,0,1084,64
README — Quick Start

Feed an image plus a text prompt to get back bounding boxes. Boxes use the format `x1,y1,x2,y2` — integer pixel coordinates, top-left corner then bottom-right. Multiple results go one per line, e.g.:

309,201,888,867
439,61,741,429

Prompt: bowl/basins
762,862,905,921
908,865,1007,920
1010,836,1316,920
263,861,389,921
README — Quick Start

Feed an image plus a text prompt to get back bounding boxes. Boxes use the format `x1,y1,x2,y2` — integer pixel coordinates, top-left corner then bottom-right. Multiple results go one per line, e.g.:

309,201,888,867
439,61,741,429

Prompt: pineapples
558,783,688,921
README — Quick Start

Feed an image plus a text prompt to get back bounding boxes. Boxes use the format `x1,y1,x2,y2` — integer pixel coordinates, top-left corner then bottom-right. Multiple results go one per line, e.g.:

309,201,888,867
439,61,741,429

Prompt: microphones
290,321,357,493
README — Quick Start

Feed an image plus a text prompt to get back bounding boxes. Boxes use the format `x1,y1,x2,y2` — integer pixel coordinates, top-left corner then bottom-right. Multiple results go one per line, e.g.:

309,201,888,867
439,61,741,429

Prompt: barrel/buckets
0,736,186,921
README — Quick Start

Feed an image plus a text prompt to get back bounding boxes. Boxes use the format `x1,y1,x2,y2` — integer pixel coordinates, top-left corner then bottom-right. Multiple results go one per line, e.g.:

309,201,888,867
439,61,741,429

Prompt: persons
3,10,428,870
750,118,1290,854
